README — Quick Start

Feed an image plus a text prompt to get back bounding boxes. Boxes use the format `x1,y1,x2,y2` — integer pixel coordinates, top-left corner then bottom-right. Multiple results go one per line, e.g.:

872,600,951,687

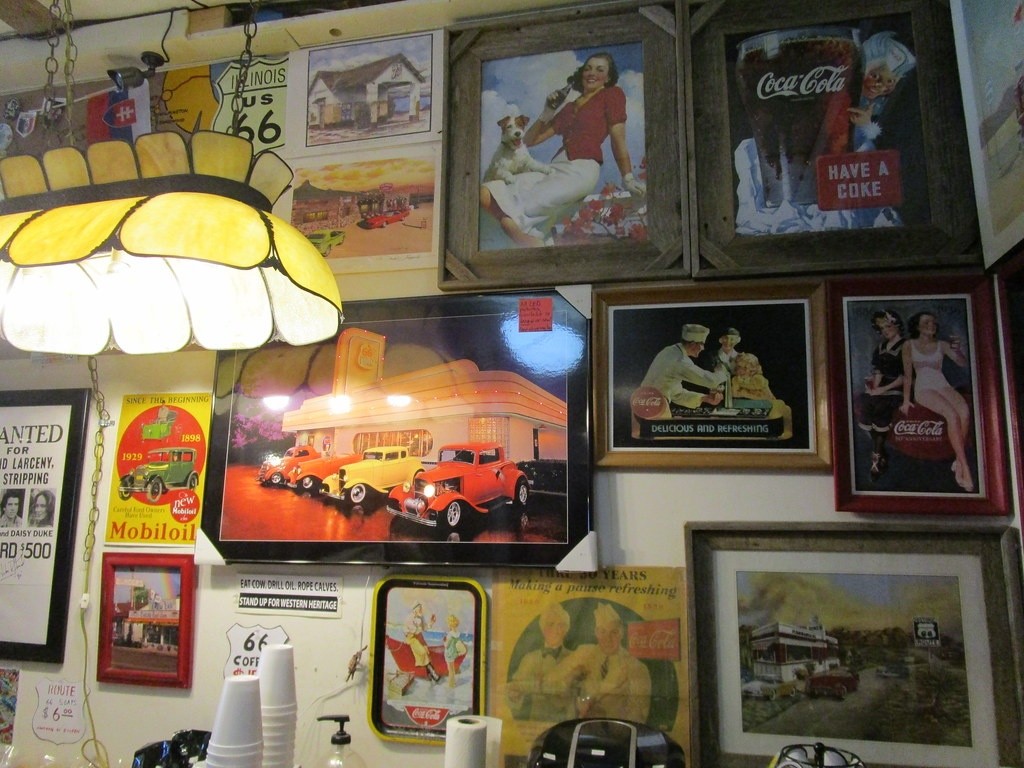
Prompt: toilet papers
441,713,504,768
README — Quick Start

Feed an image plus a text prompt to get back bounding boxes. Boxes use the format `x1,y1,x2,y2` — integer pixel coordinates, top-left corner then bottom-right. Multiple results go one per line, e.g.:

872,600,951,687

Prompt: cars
118,446,199,502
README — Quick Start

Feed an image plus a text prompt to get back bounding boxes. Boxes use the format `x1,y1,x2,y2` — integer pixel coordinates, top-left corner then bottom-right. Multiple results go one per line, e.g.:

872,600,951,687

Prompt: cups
203,638,298,768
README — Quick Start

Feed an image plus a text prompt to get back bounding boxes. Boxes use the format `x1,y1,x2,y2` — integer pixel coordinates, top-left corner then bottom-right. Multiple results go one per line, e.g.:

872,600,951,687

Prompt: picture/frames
283,27,443,160
435,0,692,294
684,520,1024,767
192,282,599,573
367,573,489,747
95,551,196,689
1,387,94,663
948,1,1023,271
590,278,834,474
681,0,986,282
273,142,442,275
827,271,1015,517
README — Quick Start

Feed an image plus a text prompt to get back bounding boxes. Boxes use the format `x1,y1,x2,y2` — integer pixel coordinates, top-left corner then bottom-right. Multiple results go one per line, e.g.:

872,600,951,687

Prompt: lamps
1,1,345,356
